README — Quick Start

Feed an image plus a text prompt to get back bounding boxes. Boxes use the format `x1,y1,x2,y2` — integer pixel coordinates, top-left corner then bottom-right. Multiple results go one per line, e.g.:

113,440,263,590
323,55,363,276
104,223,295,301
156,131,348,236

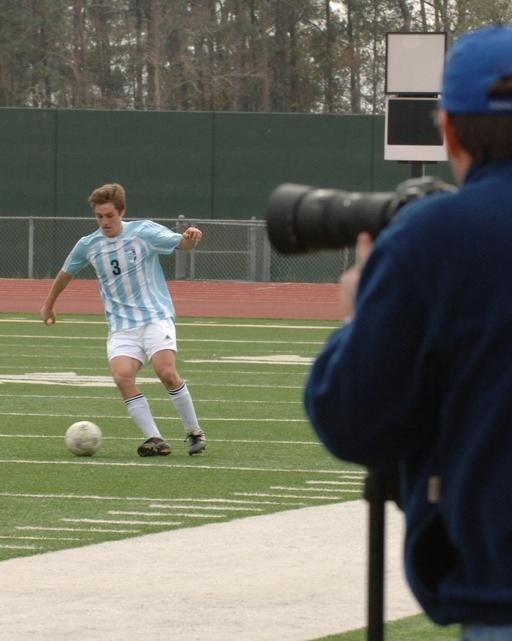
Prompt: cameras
266,175,458,256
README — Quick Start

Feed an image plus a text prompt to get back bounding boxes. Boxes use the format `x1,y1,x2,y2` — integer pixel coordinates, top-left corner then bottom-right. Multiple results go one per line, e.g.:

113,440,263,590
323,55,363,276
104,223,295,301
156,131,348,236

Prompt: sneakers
137,437,170,457
189,431,206,454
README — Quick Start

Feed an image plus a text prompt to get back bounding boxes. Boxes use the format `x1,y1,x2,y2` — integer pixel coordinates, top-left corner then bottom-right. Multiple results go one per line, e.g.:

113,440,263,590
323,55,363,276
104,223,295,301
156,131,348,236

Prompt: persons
39,180,210,460
298,20,511,641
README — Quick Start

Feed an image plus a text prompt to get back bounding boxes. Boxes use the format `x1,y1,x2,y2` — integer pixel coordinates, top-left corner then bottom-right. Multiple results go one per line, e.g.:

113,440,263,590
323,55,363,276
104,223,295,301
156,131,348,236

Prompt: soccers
66,421,102,456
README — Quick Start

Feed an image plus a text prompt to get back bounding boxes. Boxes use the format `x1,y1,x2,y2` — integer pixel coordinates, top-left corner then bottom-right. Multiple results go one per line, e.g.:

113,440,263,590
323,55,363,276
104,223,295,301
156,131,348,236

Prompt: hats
442,27,512,113
